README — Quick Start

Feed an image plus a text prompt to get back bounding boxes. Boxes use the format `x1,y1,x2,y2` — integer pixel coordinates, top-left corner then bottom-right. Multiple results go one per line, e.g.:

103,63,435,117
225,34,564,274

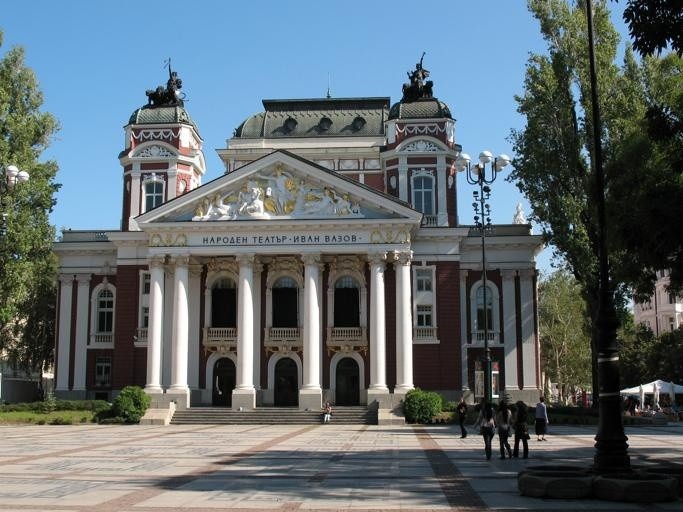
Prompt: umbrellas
620,379,683,395
653,384,660,410
639,384,645,410
669,381,676,408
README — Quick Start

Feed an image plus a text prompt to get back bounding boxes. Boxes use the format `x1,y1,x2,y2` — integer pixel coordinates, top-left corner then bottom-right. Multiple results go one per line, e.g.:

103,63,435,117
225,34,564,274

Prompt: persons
491,400,512,459
455,396,467,439
471,401,497,460
322,401,333,424
190,164,362,222
533,396,549,442
510,401,530,459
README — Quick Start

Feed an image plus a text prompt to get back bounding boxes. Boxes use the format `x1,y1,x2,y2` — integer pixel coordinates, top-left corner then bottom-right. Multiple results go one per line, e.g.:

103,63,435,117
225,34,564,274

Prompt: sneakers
537,437,547,441
485,454,527,460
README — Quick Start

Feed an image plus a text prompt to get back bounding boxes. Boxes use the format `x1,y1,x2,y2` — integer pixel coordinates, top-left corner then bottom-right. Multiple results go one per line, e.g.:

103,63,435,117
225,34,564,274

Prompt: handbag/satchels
508,426,512,437
489,423,496,434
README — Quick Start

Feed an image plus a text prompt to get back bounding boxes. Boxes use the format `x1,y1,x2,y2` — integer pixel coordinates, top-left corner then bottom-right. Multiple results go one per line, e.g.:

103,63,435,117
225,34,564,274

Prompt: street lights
0,165,29,236
455,151,511,402
